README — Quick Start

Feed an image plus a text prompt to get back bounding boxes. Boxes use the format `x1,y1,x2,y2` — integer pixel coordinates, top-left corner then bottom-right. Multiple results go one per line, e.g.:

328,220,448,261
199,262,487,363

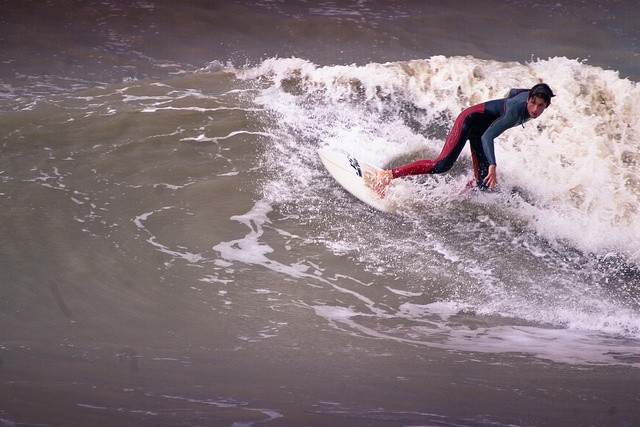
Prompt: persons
374,83,557,199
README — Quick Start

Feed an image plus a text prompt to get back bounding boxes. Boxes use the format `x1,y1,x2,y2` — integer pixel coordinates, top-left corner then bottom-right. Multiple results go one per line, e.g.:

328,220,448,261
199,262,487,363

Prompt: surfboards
318,149,403,213
